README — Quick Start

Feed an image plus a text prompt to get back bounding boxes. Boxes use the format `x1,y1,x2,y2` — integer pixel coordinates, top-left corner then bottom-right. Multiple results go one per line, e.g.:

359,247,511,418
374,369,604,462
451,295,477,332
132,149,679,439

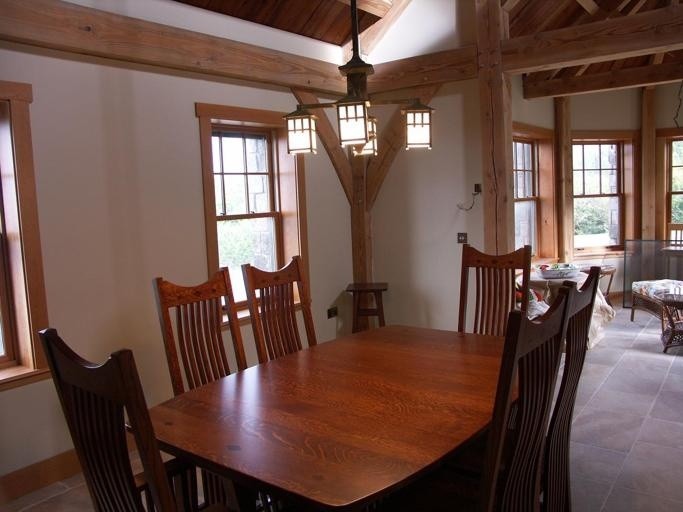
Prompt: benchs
632,279,682,334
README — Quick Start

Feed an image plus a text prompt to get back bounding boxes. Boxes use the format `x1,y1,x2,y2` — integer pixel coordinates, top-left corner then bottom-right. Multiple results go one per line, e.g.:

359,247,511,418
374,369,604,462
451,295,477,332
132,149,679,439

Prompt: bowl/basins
533,263,580,279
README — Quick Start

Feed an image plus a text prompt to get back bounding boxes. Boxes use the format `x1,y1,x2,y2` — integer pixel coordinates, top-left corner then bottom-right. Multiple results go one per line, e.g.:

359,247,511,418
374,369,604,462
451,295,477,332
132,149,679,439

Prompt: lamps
282,0,437,156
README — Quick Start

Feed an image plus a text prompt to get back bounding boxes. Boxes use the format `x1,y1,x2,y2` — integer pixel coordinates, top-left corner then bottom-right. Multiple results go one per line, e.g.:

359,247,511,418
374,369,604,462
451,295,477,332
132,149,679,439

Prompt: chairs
39,326,241,512
455,244,531,333
240,257,316,363
405,285,571,512
502,267,600,512
151,267,248,398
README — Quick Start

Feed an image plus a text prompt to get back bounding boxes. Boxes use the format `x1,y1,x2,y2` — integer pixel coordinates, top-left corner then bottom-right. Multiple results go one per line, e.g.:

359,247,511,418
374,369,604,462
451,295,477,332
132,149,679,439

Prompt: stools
347,282,387,332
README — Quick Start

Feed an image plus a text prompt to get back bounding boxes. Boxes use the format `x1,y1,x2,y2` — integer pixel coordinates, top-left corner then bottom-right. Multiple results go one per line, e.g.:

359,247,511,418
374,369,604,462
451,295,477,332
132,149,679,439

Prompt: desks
661,294,683,353
121,323,518,512
517,267,616,353
